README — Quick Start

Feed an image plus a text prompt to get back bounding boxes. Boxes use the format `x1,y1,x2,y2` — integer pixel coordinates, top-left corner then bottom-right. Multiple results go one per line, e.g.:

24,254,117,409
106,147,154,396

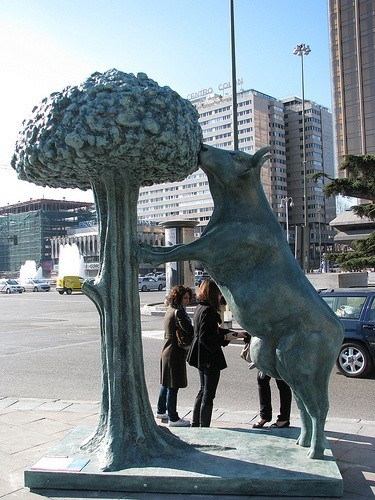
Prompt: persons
156,283,194,427
246,332,291,429
186,278,249,429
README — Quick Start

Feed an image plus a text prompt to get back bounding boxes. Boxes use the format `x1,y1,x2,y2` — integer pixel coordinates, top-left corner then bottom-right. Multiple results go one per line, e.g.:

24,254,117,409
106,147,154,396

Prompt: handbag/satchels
175,308,194,347
240,343,253,363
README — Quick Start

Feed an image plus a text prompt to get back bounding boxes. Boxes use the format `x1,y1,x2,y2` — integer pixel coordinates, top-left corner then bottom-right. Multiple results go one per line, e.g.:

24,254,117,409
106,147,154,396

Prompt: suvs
316,288,375,378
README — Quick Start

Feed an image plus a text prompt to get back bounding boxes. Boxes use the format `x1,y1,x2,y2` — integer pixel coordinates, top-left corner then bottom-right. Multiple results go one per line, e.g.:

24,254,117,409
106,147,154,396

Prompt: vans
56,276,86,295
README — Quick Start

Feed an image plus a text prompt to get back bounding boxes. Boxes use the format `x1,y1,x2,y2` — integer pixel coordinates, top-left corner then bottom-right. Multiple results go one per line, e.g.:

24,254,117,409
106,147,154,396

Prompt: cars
21,279,50,292
195,271,211,286
0,279,25,294
138,272,166,292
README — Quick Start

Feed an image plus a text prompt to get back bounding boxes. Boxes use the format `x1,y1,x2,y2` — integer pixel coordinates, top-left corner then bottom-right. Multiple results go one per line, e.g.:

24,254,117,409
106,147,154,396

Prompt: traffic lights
14,235,18,245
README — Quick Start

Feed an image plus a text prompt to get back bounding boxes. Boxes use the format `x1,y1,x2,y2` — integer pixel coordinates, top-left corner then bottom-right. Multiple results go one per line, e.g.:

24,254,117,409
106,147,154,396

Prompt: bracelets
225,334,228,341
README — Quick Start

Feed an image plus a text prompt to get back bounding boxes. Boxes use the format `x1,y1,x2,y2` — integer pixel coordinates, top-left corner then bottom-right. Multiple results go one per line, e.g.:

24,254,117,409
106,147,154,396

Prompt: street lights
314,205,323,271
280,197,295,245
292,43,311,272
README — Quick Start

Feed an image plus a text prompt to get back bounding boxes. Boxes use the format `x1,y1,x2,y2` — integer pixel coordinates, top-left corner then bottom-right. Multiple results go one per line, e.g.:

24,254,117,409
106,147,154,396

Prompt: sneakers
156,413,169,419
170,420,191,426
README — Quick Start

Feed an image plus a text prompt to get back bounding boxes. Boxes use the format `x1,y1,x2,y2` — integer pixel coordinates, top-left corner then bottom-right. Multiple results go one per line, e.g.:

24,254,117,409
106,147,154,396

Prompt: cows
136,145,344,459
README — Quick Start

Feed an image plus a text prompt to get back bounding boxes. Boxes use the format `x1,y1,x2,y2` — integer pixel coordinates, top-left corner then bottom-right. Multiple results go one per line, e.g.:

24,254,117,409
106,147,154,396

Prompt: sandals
253,419,271,428
270,421,290,428
